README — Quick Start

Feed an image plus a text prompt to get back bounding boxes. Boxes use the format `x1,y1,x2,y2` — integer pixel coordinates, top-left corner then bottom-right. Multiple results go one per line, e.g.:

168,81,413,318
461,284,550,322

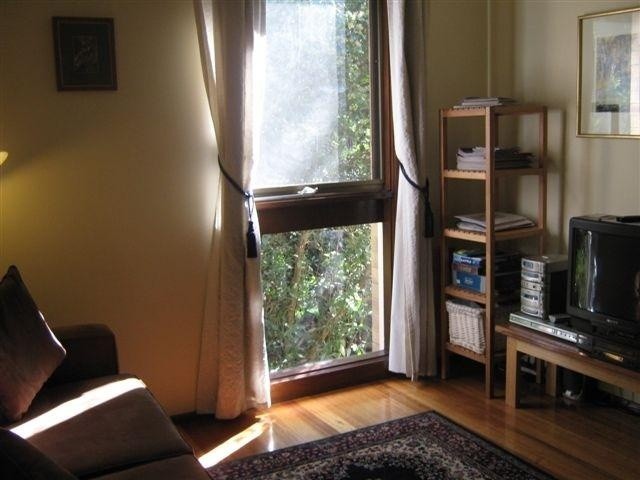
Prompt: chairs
0,322,122,428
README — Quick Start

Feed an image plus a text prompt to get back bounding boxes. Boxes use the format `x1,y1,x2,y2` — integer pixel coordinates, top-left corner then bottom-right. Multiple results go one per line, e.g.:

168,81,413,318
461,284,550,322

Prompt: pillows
1,429,72,478
3,264,66,421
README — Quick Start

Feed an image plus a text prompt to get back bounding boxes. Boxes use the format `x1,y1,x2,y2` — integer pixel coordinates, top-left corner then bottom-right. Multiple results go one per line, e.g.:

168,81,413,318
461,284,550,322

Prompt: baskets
445,300,519,355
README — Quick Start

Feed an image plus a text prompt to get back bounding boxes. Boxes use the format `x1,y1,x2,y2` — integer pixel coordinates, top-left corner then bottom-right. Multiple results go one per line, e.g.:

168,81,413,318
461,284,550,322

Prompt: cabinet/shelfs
438,105,548,399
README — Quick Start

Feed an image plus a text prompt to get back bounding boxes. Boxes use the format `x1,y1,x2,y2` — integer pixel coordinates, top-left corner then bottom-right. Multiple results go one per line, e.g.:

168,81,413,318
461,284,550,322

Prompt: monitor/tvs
568,213,640,337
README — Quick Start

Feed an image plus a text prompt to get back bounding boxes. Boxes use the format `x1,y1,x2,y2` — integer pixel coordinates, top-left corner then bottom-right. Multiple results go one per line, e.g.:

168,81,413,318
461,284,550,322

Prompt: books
456,146,535,171
461,96,518,107
454,214,535,233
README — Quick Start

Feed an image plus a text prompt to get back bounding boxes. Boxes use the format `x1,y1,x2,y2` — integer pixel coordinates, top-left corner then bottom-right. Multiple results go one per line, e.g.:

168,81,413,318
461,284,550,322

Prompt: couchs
1,324,214,480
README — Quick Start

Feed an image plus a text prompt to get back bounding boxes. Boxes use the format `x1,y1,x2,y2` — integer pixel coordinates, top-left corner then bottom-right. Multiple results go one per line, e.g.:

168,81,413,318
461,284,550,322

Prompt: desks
494,323,640,408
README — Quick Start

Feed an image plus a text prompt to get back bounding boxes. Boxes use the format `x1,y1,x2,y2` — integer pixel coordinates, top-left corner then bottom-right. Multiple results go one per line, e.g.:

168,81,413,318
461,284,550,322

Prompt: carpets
204,409,566,479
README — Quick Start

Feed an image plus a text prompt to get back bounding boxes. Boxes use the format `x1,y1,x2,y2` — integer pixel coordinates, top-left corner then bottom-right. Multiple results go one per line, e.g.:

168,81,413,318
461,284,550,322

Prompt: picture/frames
575,7,640,138
51,14,119,91
